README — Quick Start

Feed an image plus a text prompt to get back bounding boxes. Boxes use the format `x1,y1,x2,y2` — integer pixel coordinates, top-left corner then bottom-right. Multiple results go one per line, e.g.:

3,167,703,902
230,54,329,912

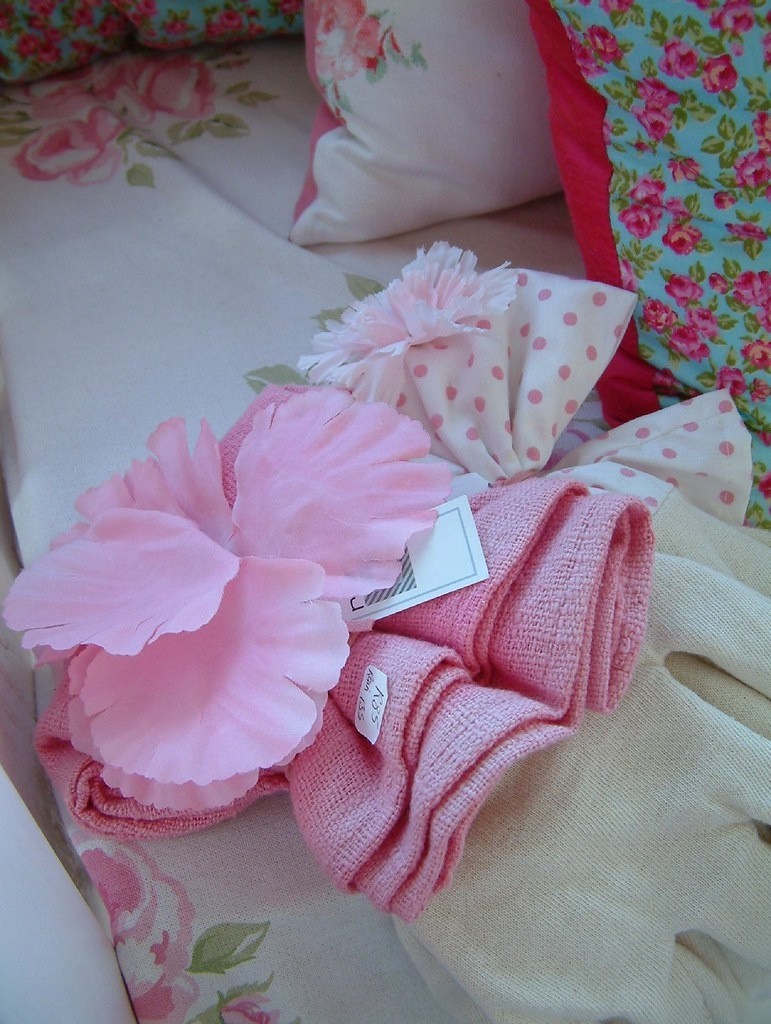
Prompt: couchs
0,0,771,1024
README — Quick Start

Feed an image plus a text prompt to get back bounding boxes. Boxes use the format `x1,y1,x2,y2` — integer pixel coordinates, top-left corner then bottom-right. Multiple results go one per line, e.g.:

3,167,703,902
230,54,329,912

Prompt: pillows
289,0,562,248
524,0,771,529
